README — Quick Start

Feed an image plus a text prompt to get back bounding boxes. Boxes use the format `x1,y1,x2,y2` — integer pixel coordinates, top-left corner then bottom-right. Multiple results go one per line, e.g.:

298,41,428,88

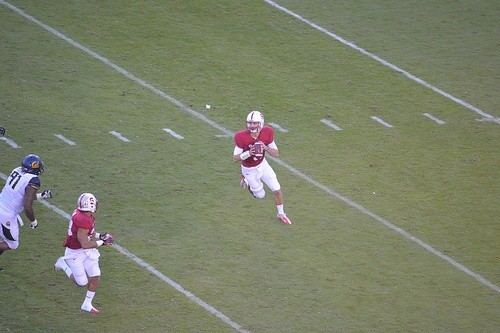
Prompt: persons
0,154,53,271
233,111,291,225
54,192,113,313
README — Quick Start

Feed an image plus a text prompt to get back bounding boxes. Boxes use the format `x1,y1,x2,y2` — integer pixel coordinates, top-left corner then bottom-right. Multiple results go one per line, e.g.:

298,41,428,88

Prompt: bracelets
241,151,251,160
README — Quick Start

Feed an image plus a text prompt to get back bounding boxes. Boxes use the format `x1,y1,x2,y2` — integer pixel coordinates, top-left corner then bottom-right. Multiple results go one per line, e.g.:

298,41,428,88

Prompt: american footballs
254,140,264,161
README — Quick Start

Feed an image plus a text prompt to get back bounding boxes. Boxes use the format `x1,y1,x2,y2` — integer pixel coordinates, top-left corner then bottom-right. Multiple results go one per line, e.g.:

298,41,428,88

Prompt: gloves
36,190,53,200
95,234,113,248
30,220,38,229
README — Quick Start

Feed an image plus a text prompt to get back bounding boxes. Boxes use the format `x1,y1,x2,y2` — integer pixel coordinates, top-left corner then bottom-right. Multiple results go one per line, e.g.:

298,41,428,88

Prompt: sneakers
277,213,292,225
54,257,65,272
241,174,248,189
81,305,100,314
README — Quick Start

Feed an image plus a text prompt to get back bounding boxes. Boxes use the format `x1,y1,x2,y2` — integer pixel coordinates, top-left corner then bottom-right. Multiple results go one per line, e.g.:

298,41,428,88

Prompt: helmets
77,193,99,212
247,111,264,133
20,155,44,176
